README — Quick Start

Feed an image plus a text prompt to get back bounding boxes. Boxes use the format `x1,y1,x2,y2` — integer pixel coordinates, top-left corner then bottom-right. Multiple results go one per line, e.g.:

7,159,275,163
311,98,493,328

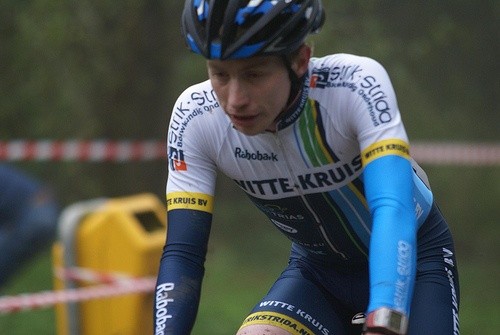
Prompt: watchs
365,306,409,335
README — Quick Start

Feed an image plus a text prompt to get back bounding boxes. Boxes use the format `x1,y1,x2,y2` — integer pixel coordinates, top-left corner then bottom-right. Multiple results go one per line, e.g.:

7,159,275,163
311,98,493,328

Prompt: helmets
181,0,326,61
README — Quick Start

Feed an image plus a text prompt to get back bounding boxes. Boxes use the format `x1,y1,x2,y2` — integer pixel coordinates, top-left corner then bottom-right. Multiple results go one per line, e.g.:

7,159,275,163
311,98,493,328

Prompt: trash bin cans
51,192,169,335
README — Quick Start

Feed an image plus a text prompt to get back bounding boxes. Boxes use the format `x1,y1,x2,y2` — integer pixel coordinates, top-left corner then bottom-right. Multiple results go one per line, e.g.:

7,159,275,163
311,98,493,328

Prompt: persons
0,161,57,288
152,0,461,335
